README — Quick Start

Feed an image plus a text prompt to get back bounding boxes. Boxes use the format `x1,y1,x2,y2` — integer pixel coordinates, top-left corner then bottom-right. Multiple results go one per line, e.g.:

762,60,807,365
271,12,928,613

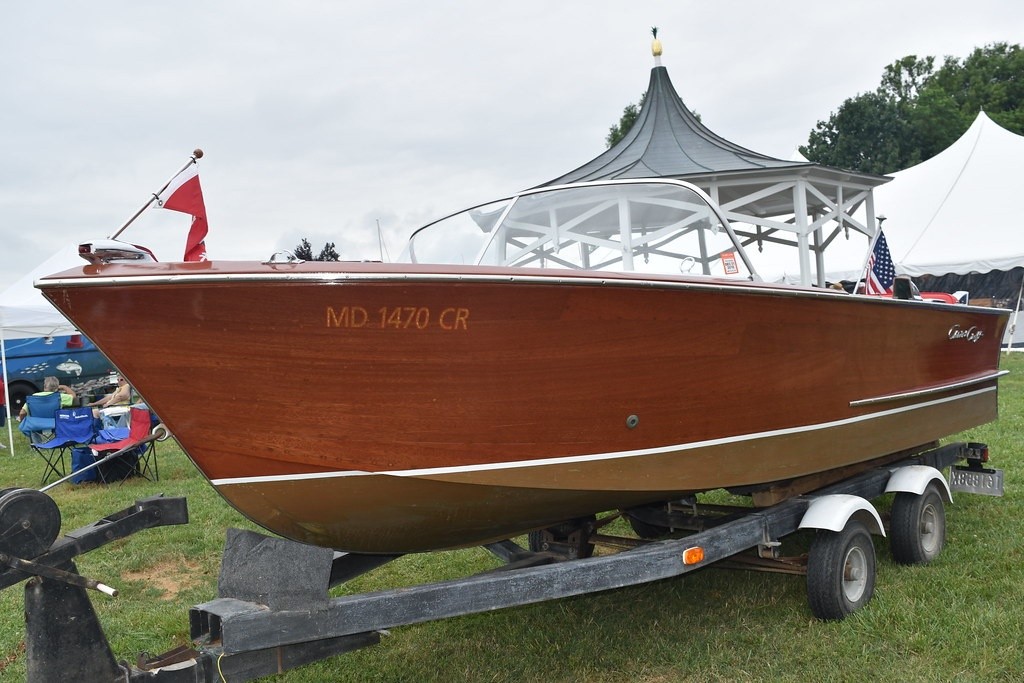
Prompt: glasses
117,379,124,381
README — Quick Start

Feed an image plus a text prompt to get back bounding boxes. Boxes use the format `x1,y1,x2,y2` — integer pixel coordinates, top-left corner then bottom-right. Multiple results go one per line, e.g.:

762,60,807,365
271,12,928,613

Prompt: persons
19,376,76,421
858,282,866,294
88,372,130,418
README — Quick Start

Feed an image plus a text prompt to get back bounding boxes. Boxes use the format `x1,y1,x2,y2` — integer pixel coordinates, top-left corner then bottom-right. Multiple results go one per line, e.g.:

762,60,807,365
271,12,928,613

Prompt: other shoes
0,443,6,449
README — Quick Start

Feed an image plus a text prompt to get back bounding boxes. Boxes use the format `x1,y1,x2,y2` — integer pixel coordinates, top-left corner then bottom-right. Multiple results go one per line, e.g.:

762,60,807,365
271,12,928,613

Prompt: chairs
20,392,159,491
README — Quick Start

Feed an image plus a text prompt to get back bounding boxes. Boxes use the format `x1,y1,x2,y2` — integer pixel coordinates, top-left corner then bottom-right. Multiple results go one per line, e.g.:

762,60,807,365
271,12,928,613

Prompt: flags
153,162,209,261
865,230,897,295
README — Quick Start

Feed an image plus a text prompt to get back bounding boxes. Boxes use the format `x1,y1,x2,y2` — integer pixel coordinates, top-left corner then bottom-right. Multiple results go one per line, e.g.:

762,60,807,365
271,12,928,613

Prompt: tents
504,103,1023,357
0,235,156,458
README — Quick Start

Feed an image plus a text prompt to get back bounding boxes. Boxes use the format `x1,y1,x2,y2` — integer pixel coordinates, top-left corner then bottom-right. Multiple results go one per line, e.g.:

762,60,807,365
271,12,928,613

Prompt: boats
33,26,1014,556
